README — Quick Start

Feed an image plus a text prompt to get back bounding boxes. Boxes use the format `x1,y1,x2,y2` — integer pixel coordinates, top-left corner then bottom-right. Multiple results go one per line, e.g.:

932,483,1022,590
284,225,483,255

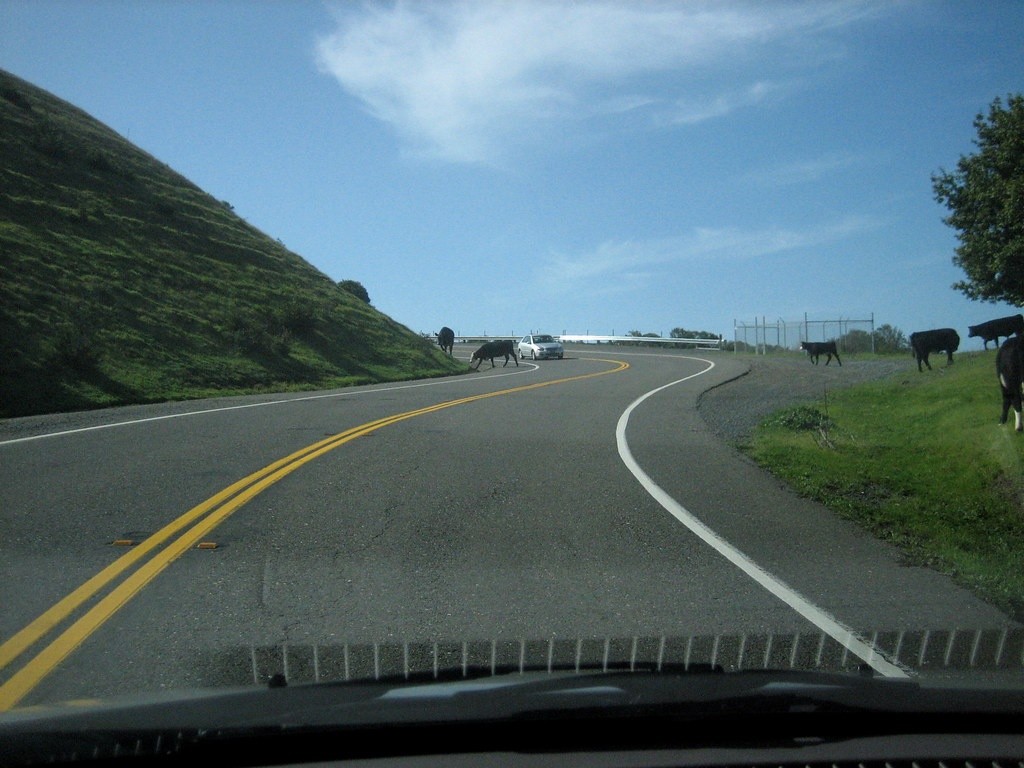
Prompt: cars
518,334,564,360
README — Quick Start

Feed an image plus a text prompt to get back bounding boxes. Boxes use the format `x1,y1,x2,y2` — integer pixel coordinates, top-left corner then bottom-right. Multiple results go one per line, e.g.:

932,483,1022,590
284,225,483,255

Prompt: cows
968,313,1024,350
909,328,960,371
800,340,843,367
434,326,455,356
470,340,519,371
996,337,1024,433
